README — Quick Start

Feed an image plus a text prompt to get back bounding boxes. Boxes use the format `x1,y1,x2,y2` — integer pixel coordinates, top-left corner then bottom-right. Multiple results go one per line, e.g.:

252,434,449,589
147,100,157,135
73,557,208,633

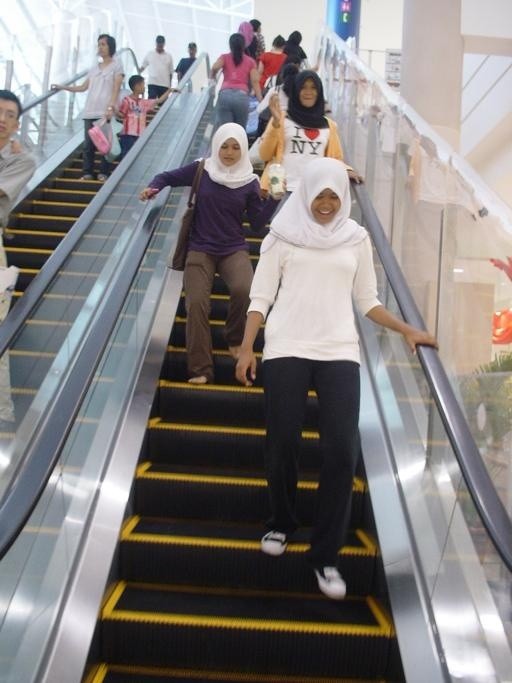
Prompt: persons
0,90,37,433
139,123,287,384
50,32,198,180
236,156,440,601
207,20,307,139
248,69,363,198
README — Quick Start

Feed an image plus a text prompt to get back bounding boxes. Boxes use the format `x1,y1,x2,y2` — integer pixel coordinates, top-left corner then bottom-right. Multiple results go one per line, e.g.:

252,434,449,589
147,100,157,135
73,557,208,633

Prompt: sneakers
262,531,287,557
314,565,346,599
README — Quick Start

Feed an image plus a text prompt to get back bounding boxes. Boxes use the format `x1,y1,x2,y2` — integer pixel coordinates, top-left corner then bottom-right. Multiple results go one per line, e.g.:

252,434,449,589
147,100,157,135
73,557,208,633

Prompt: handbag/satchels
257,106,276,137
88,126,110,154
172,206,194,271
